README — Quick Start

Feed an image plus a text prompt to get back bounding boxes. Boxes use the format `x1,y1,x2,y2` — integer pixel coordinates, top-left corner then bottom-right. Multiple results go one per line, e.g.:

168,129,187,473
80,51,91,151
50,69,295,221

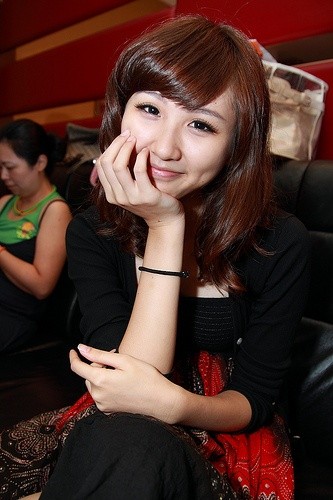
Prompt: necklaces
13,184,57,216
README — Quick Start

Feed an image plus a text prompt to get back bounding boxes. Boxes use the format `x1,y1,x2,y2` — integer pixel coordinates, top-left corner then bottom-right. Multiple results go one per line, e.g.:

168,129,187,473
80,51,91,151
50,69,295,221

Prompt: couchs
1,154,333,500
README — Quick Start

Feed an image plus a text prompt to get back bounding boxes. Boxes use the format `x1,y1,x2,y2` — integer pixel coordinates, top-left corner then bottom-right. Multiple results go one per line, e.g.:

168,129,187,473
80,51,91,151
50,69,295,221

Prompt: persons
19,15,333,500
0,120,74,376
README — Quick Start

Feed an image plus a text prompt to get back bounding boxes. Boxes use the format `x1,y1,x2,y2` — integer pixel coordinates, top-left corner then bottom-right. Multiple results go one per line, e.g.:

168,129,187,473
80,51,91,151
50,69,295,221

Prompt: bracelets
0,247,6,253
138,265,189,278
